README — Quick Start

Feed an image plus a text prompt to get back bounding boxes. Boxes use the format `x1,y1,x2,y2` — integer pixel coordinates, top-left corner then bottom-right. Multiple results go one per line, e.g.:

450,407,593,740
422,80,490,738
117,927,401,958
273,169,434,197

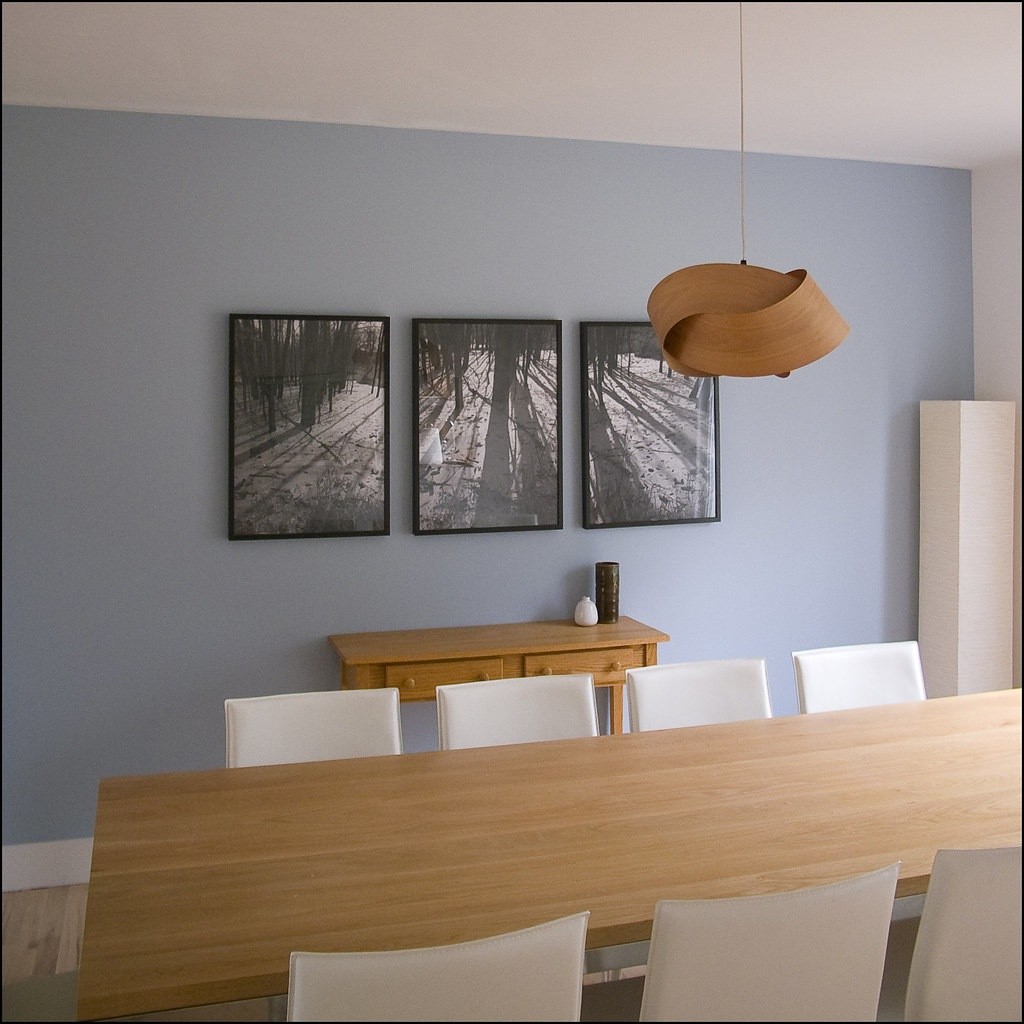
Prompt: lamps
647,2,849,378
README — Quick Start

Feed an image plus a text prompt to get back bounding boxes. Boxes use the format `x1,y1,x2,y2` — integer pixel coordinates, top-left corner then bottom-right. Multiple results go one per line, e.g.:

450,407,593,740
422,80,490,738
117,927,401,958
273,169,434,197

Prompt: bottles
595,562,619,624
574,595,599,626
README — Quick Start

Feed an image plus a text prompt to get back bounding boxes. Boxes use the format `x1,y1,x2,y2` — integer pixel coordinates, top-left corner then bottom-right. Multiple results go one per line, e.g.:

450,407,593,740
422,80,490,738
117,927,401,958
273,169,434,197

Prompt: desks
327,615,670,734
77,687,1022,1022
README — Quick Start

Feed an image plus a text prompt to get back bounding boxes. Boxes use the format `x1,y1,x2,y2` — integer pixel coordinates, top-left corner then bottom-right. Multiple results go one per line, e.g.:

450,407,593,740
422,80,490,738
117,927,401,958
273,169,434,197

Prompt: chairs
436,673,600,751
581,860,901,1022
790,641,926,715
625,657,773,732
288,909,591,1022
224,687,401,768
877,846,1021,1022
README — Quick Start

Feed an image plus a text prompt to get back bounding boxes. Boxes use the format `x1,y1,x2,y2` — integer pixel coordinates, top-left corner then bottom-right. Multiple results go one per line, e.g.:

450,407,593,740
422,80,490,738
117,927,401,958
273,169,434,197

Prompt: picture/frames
412,318,564,537
579,321,721,530
228,313,390,541
